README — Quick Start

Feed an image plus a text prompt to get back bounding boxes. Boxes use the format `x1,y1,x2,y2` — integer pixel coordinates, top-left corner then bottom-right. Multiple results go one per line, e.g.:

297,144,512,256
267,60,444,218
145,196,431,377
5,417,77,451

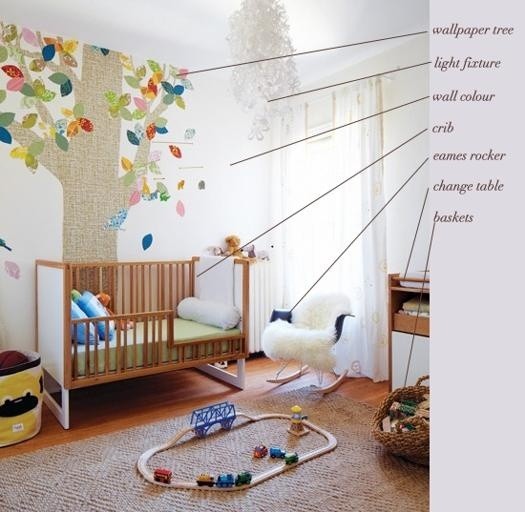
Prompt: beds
34,253,249,430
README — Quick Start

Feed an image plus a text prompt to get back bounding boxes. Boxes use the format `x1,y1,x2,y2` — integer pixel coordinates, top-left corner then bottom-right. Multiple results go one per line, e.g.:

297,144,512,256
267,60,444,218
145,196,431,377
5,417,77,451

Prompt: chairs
261,292,355,395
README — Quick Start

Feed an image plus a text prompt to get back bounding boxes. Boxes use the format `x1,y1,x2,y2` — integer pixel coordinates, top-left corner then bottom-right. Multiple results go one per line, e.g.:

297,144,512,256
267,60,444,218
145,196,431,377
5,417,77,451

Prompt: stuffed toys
95,292,135,332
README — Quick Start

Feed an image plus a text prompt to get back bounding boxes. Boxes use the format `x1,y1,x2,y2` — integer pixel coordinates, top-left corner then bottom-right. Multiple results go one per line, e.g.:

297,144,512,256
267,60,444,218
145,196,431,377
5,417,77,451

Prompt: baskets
371,375,430,466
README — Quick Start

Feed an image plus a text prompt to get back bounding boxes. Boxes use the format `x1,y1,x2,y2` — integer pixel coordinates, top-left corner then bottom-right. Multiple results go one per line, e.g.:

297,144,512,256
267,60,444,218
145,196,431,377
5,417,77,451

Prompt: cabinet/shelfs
386,274,429,392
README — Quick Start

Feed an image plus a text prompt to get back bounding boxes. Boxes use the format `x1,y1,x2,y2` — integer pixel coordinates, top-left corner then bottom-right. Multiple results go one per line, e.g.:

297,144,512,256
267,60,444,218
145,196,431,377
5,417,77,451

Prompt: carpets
0,383,429,512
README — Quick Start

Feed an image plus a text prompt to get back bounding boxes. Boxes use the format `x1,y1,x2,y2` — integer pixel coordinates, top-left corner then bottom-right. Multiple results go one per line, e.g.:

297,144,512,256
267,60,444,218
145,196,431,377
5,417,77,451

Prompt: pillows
71,299,100,345
79,289,116,340
176,296,241,328
